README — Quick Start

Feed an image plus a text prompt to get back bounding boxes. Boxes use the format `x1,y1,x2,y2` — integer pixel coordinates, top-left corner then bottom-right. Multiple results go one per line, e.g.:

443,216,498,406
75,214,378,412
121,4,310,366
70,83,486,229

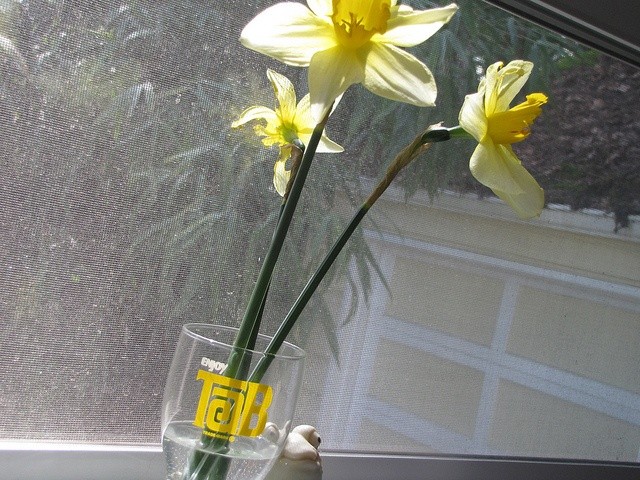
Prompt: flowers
187,1,548,480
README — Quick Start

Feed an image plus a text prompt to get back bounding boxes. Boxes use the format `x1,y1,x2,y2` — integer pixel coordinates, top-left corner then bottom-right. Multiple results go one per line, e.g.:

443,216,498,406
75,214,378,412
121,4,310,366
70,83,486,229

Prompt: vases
160,324,305,480
